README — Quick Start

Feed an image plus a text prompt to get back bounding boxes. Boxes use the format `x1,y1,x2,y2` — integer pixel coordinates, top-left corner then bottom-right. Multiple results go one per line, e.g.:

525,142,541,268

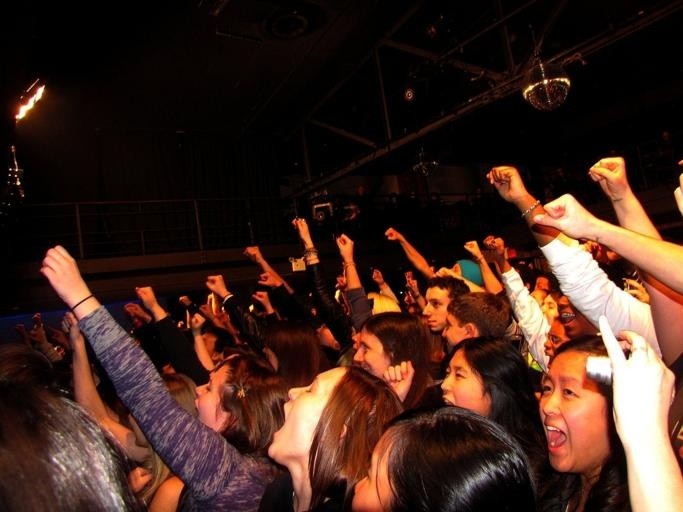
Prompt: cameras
587,354,612,384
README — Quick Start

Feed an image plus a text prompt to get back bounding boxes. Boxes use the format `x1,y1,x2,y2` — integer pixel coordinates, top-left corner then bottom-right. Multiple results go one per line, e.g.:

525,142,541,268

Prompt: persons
0,154,683,511
